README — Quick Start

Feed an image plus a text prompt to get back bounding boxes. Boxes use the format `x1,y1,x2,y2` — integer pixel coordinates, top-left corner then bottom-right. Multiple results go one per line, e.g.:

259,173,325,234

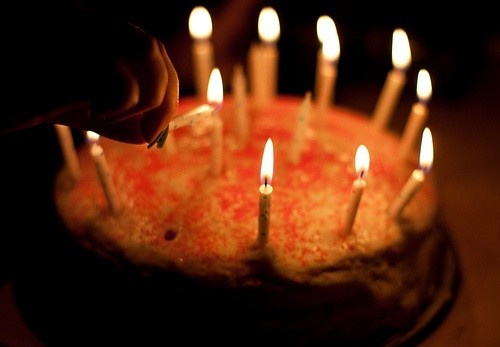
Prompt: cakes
50,92,444,345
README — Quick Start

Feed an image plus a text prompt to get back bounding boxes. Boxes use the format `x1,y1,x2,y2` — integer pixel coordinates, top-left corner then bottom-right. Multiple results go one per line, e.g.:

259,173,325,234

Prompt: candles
54,3,435,244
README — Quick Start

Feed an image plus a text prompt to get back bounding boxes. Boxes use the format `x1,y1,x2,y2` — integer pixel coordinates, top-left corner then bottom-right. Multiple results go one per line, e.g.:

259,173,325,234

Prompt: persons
0,1,180,150
